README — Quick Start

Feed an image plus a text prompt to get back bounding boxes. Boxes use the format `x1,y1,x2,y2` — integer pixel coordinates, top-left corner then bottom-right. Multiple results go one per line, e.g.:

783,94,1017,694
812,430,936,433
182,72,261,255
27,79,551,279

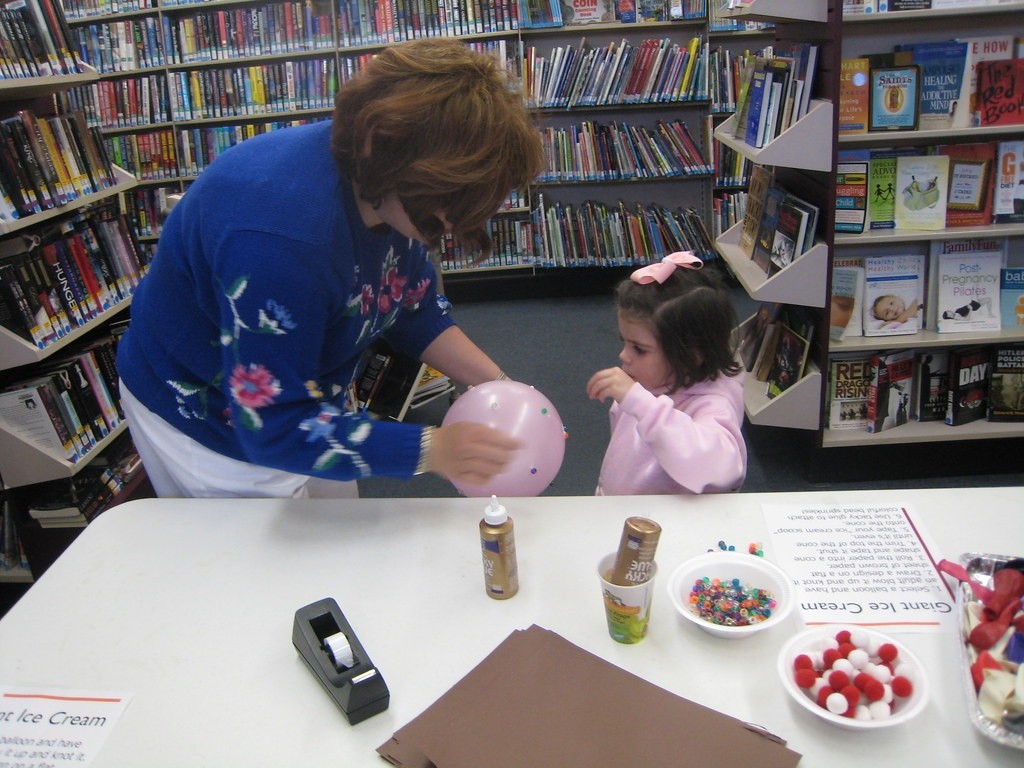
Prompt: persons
113,37,570,501
873,296,922,331
586,251,748,496
828,268,857,344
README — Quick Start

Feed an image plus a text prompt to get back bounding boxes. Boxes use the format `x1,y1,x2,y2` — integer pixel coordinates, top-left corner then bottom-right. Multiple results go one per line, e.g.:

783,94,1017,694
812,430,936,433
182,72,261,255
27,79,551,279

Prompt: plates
957,550,1024,749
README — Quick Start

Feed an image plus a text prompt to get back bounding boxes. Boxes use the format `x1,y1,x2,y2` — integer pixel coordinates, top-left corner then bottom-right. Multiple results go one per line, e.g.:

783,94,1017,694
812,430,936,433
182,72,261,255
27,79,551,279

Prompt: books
0,0,1024,575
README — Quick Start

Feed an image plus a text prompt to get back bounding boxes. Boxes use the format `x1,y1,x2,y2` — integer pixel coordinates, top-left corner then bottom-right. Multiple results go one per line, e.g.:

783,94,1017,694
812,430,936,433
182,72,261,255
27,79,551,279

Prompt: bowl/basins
775,623,933,731
666,550,799,641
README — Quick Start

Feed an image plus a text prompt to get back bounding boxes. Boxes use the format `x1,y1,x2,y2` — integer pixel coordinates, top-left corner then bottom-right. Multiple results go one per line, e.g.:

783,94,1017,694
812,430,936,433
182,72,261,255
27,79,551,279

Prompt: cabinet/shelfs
66,0,776,274
713,0,1024,484
0,0,139,584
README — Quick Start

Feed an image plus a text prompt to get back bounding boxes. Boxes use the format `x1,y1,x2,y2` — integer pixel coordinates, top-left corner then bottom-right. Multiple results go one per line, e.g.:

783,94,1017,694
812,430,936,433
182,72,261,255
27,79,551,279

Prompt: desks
0,487,1024,768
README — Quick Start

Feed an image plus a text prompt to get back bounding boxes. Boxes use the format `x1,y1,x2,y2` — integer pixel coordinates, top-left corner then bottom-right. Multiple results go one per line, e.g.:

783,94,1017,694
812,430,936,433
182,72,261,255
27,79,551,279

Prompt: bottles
479,492,519,601
611,517,662,586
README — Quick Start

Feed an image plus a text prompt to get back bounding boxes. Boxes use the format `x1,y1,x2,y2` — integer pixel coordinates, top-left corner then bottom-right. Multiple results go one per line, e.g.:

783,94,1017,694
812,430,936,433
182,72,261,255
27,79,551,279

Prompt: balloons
937,559,1024,726
438,381,568,496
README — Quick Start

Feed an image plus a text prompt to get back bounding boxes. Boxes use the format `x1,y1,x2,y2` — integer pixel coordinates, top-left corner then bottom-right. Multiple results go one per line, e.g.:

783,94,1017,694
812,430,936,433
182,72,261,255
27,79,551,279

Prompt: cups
598,550,659,644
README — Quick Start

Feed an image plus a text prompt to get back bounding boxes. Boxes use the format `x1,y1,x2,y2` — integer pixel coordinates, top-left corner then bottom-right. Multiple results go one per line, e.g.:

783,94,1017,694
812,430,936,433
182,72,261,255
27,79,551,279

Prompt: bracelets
412,426,434,475
497,372,507,382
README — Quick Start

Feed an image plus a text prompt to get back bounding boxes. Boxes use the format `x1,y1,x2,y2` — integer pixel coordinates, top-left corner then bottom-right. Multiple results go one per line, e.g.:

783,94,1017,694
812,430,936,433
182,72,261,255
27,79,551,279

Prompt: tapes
323,632,372,674
